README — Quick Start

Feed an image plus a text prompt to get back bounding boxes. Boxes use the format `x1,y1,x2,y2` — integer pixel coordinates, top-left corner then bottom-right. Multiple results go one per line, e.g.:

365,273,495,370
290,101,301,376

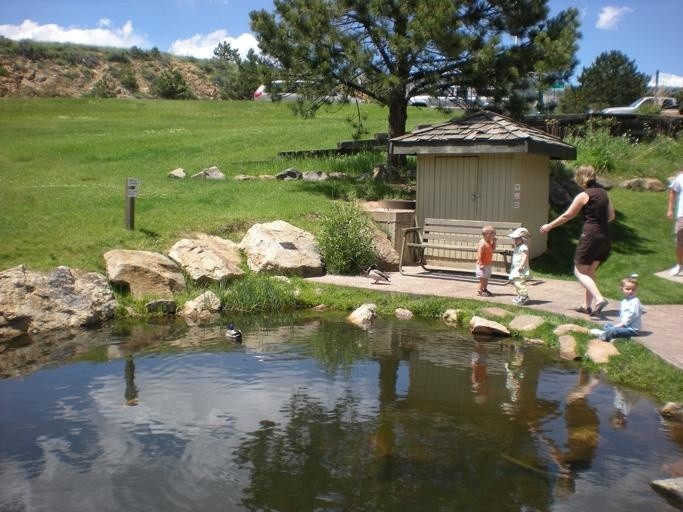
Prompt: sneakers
518,296,529,305
512,298,519,302
673,267,683,275
592,329,606,336
476,290,490,296
484,289,493,296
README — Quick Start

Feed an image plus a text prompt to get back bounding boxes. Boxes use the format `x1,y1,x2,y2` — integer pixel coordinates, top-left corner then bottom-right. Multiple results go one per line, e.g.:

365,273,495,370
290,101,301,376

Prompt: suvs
254,79,361,103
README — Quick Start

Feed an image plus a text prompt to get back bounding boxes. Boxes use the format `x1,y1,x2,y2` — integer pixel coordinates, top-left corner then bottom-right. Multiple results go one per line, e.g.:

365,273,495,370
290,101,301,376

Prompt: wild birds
225,323,242,339
367,264,392,285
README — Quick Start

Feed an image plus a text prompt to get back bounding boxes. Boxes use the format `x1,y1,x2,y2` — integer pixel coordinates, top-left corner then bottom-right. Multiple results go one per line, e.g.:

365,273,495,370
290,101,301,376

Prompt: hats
507,227,531,239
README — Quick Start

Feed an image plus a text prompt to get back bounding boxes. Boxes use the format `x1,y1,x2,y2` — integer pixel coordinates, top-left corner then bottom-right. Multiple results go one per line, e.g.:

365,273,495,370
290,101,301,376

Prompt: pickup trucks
601,96,682,116
405,82,510,109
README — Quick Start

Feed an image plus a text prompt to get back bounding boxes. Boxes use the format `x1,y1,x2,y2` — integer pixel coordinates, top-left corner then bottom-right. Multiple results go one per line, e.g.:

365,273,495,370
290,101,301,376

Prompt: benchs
397,217,523,288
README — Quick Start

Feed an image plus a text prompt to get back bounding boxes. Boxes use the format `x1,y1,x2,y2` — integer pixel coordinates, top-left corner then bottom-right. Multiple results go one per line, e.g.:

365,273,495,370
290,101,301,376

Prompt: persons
475,225,495,297
504,227,530,305
539,163,612,315
466,337,636,497
590,273,641,340
665,171,682,280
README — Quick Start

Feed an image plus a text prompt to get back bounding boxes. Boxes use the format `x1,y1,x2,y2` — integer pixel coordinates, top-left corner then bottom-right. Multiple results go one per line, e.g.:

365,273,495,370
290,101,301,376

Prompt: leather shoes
575,305,591,314
590,298,608,316
